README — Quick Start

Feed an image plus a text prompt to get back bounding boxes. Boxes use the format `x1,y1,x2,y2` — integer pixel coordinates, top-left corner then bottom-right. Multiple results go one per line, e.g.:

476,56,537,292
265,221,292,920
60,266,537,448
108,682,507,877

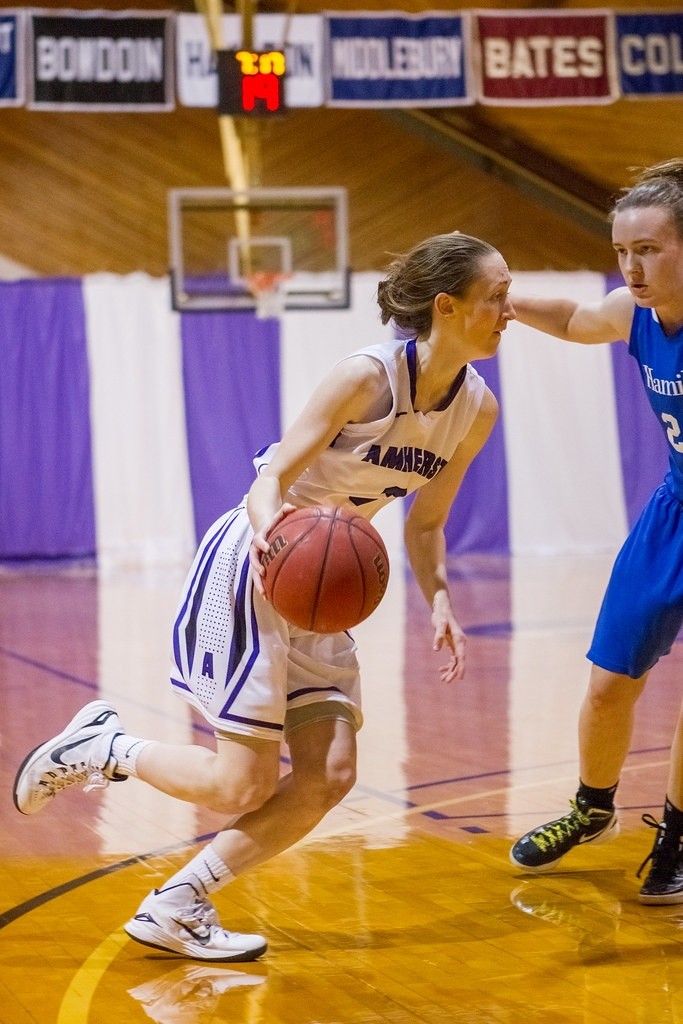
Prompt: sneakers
511,881,621,944
508,792,619,871
636,814,683,905
126,958,269,1023
13,701,127,816
124,882,267,962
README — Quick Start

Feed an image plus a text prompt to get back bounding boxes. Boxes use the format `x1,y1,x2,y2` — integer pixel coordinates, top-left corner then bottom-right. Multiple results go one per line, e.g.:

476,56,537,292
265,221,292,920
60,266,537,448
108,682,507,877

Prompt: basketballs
259,504,391,633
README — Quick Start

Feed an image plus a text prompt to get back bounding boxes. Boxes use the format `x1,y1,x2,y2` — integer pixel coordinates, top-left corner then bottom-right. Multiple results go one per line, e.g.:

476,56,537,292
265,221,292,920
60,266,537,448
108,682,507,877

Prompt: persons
12,235,517,963
507,162,683,905
